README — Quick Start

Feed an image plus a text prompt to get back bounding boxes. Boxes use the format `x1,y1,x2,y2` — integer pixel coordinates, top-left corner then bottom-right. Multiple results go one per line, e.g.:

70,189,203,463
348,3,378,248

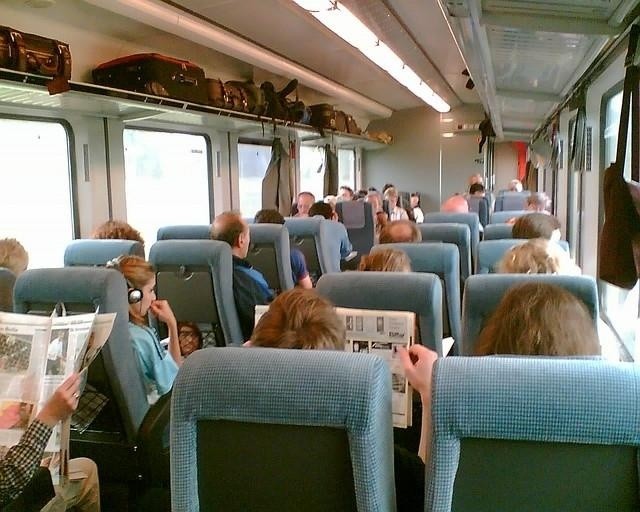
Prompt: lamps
296,1,450,114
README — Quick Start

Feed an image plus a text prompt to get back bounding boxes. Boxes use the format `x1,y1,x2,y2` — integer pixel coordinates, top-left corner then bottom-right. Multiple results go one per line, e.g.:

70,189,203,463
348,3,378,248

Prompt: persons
1,238,29,273
0,370,104,510
241,290,439,512
206,211,273,333
104,256,187,398
437,173,586,275
178,325,201,355
88,222,147,252
471,281,603,357
11,401,33,429
252,183,426,290
45,330,65,378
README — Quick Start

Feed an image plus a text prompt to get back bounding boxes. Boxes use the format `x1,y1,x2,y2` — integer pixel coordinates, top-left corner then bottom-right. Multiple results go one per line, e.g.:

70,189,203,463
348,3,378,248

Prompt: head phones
111,258,143,305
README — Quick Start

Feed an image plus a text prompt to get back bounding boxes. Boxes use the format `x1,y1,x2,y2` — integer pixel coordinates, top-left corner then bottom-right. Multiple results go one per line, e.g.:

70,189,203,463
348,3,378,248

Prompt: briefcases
0,25,72,86
93,53,362,135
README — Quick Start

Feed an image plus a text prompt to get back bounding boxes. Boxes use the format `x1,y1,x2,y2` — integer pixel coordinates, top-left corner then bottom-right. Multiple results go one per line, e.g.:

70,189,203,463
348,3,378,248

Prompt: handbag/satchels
479,119,496,137
599,164,638,290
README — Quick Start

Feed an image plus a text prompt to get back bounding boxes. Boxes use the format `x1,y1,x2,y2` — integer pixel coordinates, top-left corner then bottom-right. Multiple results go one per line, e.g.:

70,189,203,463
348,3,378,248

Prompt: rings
71,392,81,398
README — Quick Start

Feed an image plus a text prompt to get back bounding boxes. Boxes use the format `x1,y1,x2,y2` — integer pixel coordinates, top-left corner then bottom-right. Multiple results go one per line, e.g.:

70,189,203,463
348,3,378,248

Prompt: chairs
314,271,444,448
13,266,173,481
460,273,603,361
425,356,639,512
169,348,396,512
62,194,408,352
368,193,569,358
